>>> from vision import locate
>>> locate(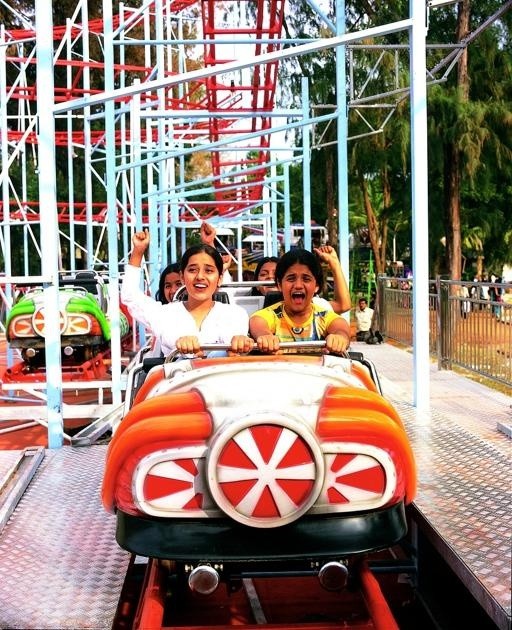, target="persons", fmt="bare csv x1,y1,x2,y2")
120,228,171,337
390,261,511,324
354,298,375,341
241,247,248,257
248,248,351,354
222,252,232,282
159,221,216,301
252,257,279,294
155,245,253,364
252,246,352,313
